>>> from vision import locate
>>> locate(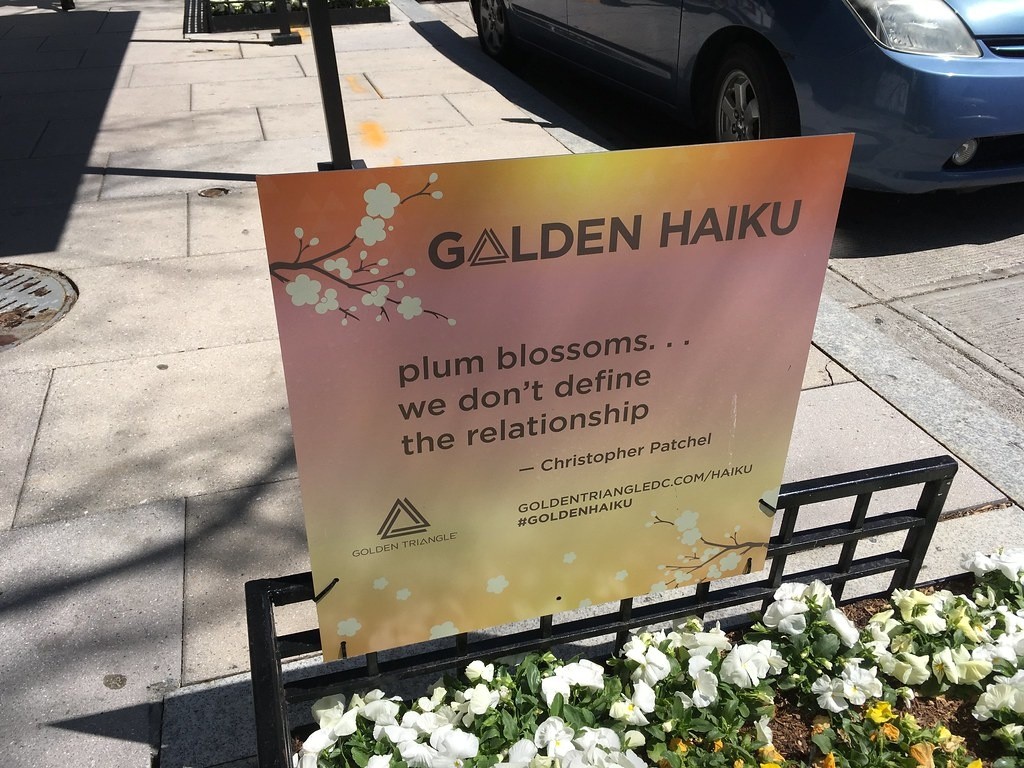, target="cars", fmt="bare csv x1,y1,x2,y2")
460,0,1022,197
